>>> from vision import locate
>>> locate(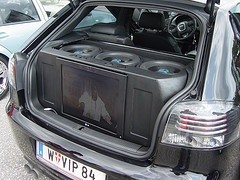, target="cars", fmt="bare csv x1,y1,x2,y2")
0,0,239,180
